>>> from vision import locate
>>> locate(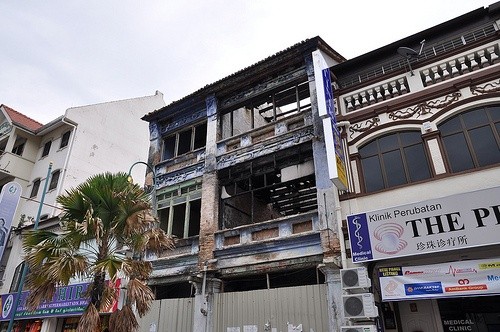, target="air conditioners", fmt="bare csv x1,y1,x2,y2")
341,292,378,321
340,324,377,332
340,266,372,291
